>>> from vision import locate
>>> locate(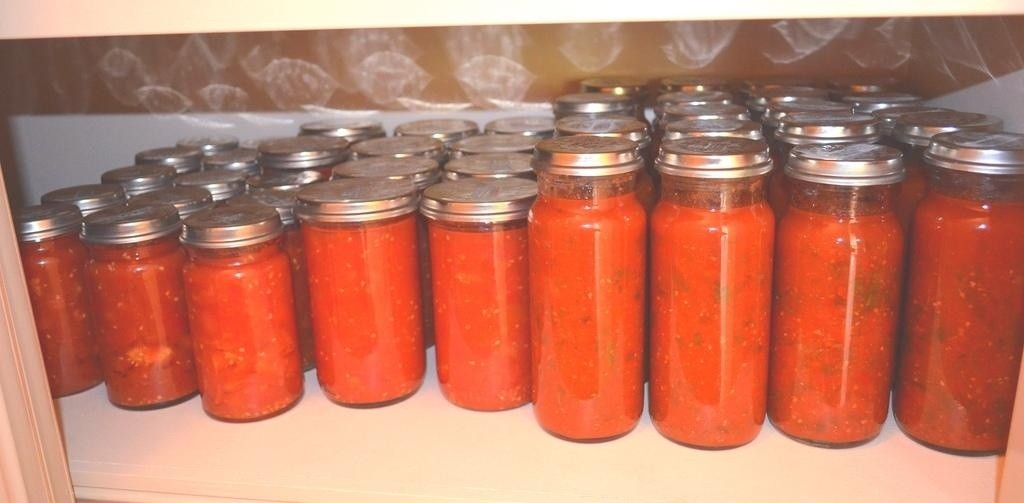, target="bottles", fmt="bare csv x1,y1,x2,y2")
12,118,552,422
527,79,1023,458
